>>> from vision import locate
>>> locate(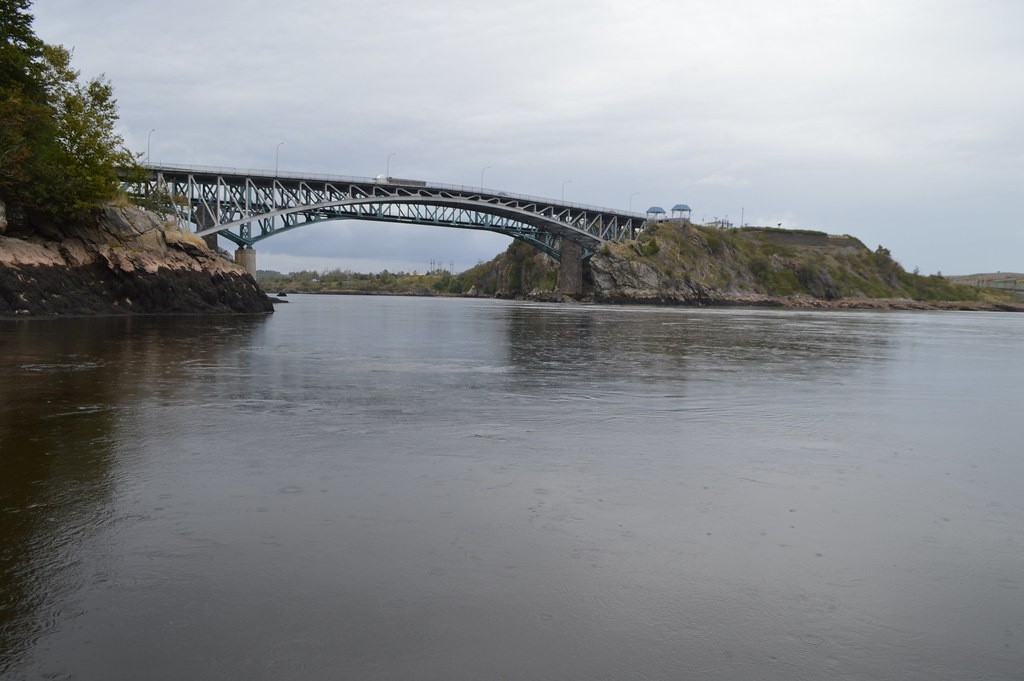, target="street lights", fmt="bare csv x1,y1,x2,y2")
148,129,154,168
387,152,395,185
276,142,284,178
480,166,491,193
562,180,571,207
